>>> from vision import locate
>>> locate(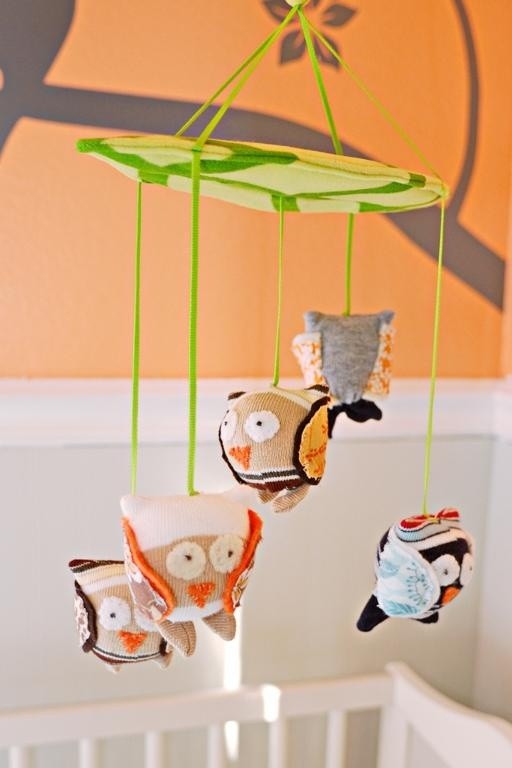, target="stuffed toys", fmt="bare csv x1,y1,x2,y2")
218,382,329,512
291,306,395,439
357,505,471,634
118,490,260,656
69,556,176,671
